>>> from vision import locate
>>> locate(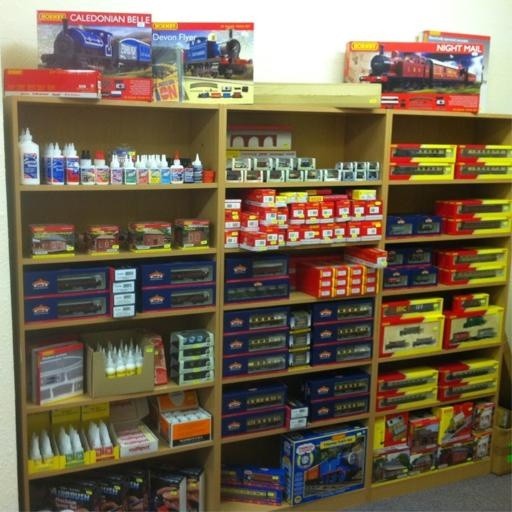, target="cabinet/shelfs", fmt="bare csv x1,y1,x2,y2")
9,94,223,512
218,101,390,512
368,108,511,499
